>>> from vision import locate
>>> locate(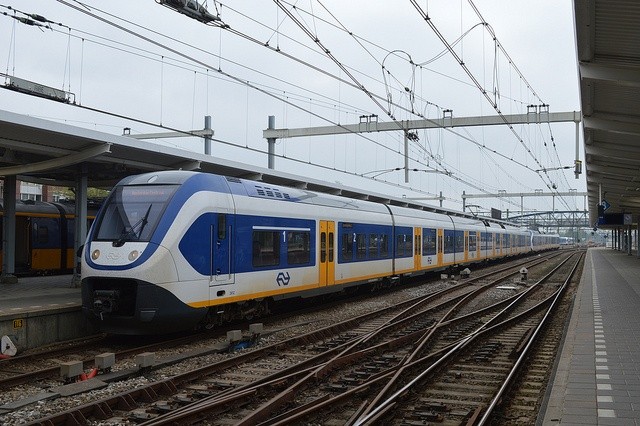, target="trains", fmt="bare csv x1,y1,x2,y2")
1,196,107,276
76,170,561,344
